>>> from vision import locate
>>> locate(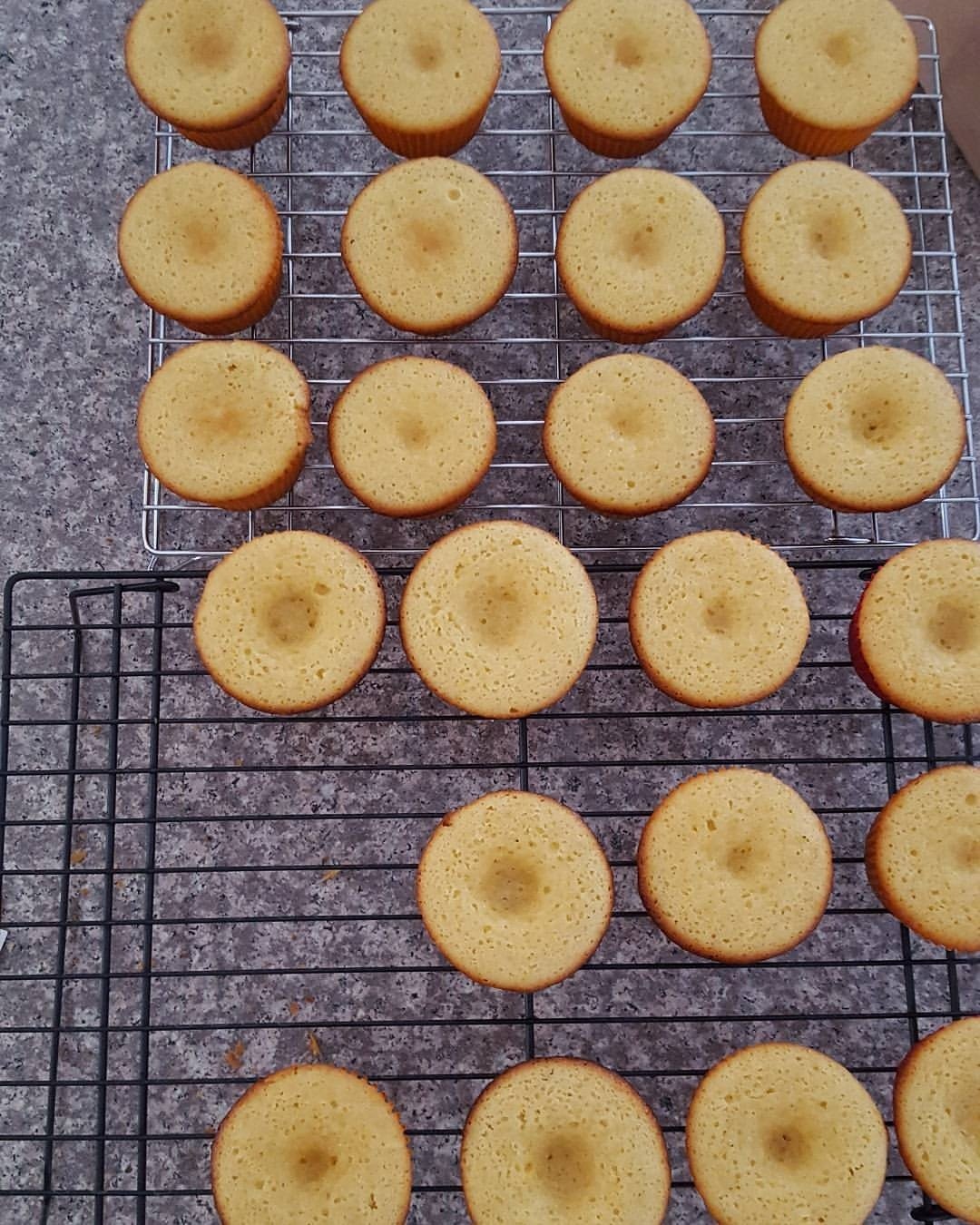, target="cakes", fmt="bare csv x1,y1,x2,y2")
117,0,975,1225
